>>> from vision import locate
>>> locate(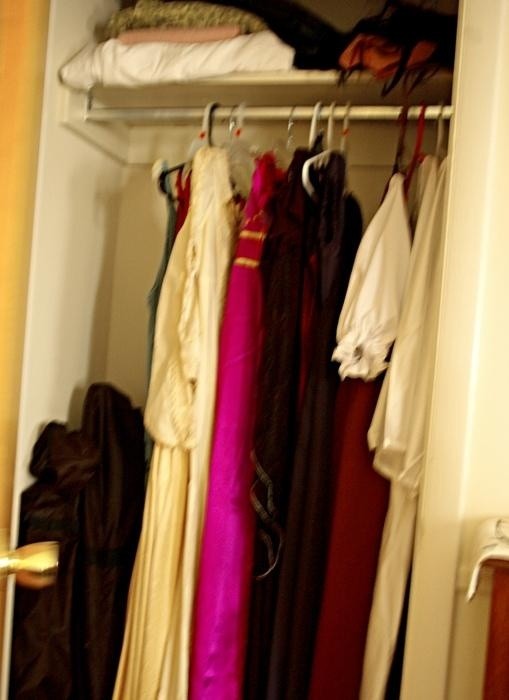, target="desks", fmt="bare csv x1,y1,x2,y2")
478,555,509,700
